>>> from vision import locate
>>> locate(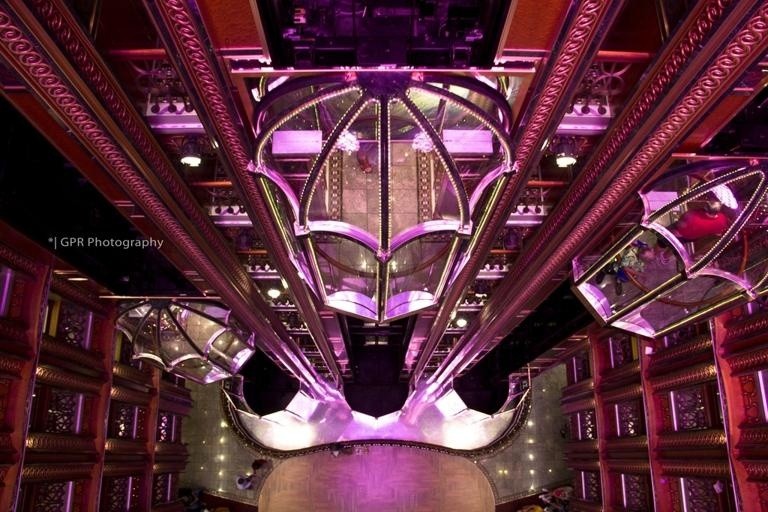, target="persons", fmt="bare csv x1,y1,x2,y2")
655,201,727,248
597,241,655,296
252,458,266,475
236,474,256,490
